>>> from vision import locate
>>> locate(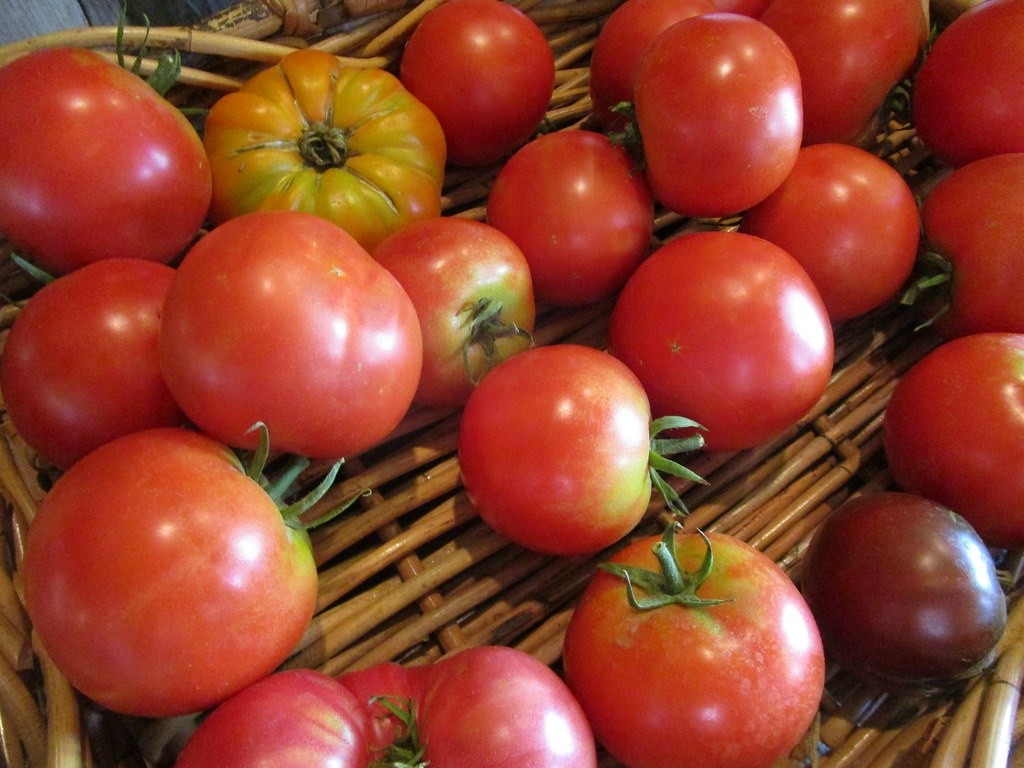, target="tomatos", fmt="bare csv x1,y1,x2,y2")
0,0,1024,768
